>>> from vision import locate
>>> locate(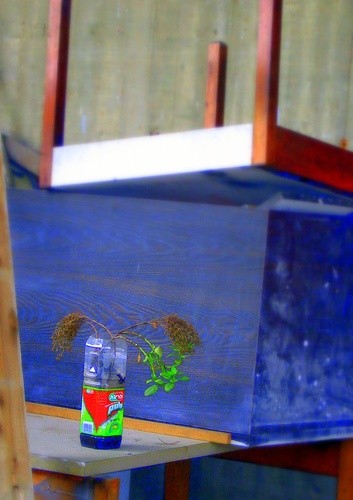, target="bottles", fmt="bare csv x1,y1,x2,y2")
77,334,128,449
